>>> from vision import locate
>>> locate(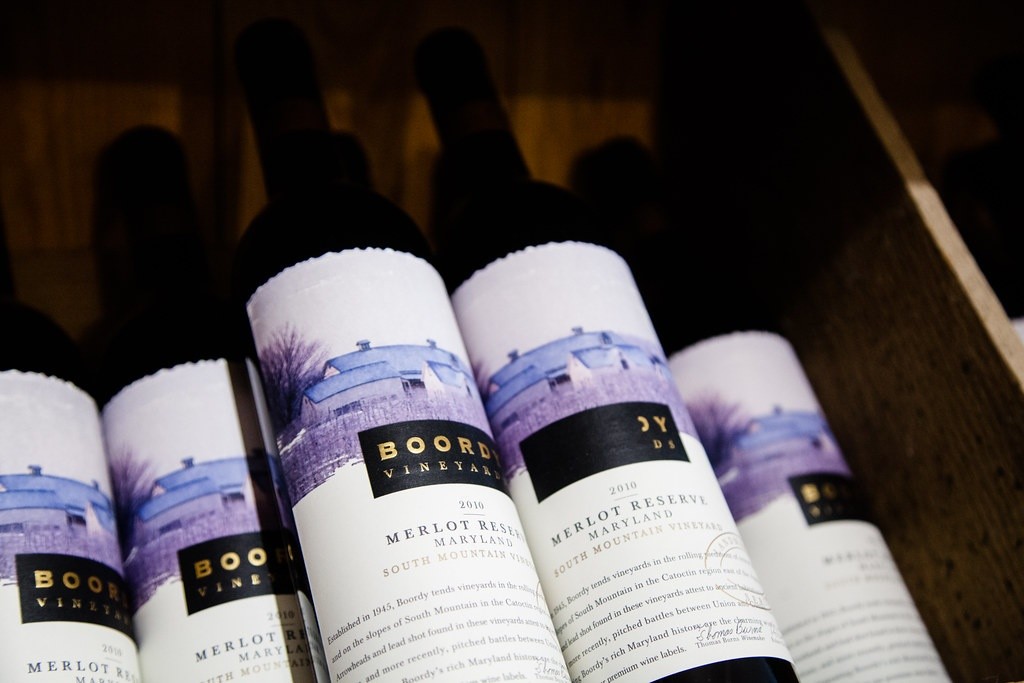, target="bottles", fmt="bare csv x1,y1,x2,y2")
0,226,135,683
92,126,329,683
226,16,547,683
413,25,794,683
599,135,954,683
